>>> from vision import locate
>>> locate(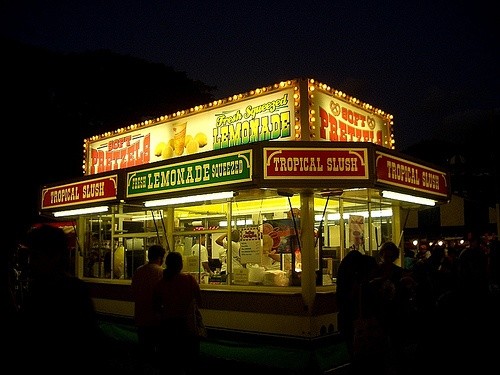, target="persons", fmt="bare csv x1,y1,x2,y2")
464,237,489,296
191,235,214,284
0,226,98,336
216,231,243,274
352,242,404,375
127,246,205,375
